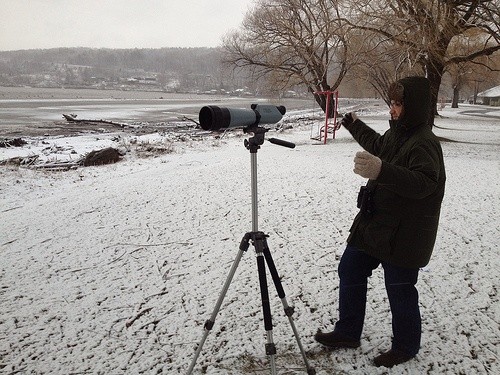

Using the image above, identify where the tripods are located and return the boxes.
[186,128,314,375]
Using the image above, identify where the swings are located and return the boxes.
[309,94,342,142]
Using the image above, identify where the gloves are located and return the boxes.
[352,150,382,180]
[340,111,359,129]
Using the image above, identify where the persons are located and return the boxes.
[313,76,447,368]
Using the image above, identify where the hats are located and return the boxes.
[387,75,439,107]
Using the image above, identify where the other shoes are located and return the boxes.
[373,349,412,367]
[314,330,361,348]
